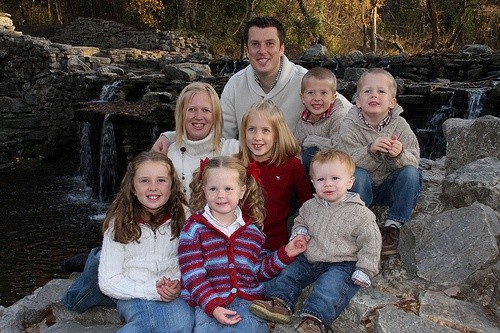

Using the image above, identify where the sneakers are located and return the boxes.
[382,224,401,255]
[249,298,290,324]
[294,315,334,333]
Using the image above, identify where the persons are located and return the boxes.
[165,83,240,203]
[151,16,355,155]
[178,156,310,333]
[339,68,420,254]
[249,149,382,333]
[98,152,194,333]
[294,66,346,172]
[232,100,312,250]
